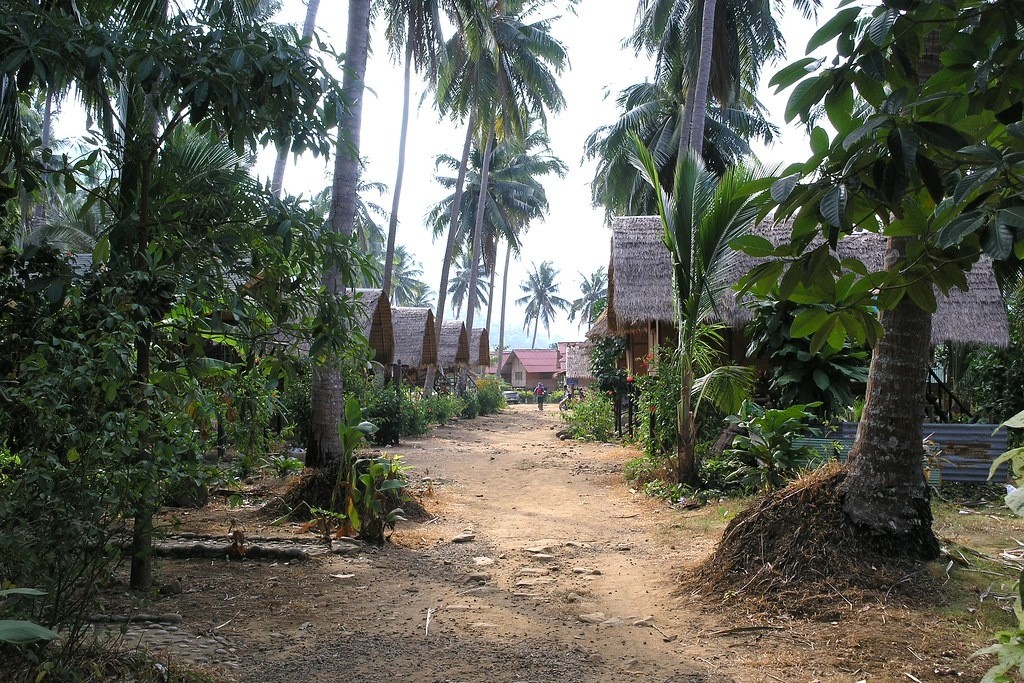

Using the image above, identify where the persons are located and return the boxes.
[563,385,577,400]
[533,382,546,411]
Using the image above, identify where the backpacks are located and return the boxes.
[537,387,543,395]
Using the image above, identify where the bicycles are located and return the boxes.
[559,385,586,412]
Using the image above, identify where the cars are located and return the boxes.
[503,391,520,404]
